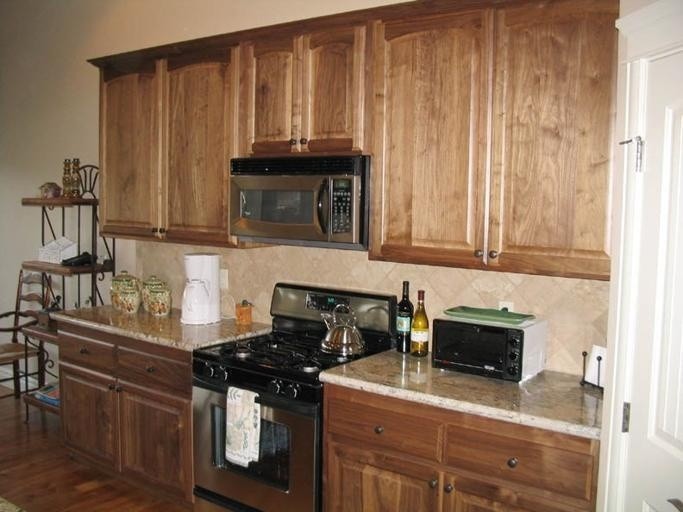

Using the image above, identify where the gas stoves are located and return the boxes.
[191,331,379,403]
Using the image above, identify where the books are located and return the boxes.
[35,380,60,407]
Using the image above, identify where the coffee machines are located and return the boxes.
[180,252,221,324]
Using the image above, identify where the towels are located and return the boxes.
[224,385,262,470]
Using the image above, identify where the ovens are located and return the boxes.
[190,373,321,512]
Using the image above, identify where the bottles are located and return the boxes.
[61,159,70,199]
[409,290,429,357]
[110,269,171,317]
[395,279,414,354]
[408,358,428,395]
[238,299,252,325]
[70,158,80,198]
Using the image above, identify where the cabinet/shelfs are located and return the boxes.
[320,381,596,512]
[85,31,281,248]
[57,318,194,503]
[366,2,608,280]
[237,8,364,159]
[20,184,117,429]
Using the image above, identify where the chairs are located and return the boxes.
[0,267,51,403]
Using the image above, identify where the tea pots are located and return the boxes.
[319,304,367,354]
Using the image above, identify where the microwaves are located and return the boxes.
[431,305,545,384]
[229,153,371,253]
